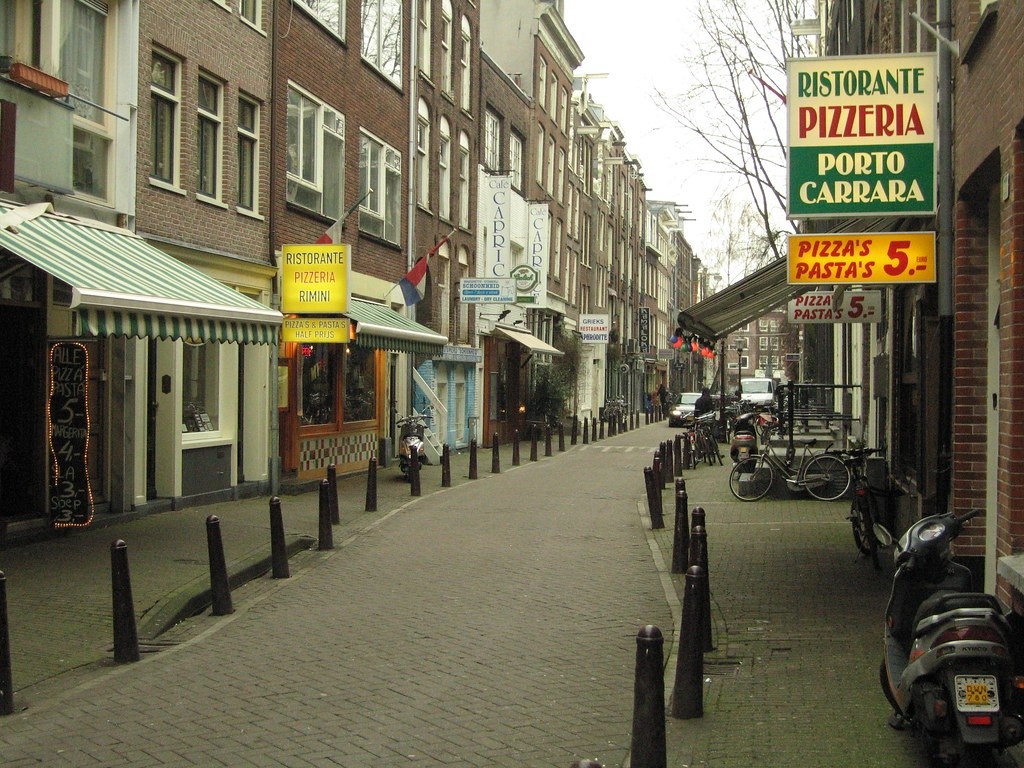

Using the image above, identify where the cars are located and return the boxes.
[667,391,702,428]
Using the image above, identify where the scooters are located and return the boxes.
[392,404,435,484]
[870,508,1024,768]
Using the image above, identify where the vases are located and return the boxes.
[0,54,70,99]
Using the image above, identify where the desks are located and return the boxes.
[770,418,840,460]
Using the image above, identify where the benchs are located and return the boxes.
[847,436,857,443]
[739,444,771,497]
[830,426,840,431]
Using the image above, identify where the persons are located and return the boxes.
[692,386,713,426]
[657,384,667,406]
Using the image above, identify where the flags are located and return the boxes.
[314,195,366,246]
[398,237,448,306]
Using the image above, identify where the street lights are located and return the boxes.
[737,348,743,401]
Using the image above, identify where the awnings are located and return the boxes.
[0,203,283,347]
[679,218,906,343]
[493,327,564,357]
[341,297,448,357]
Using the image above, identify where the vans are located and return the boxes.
[735,377,775,407]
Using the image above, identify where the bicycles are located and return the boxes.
[602,395,630,422]
[823,439,885,570]
[681,394,788,473]
[727,413,852,502]
